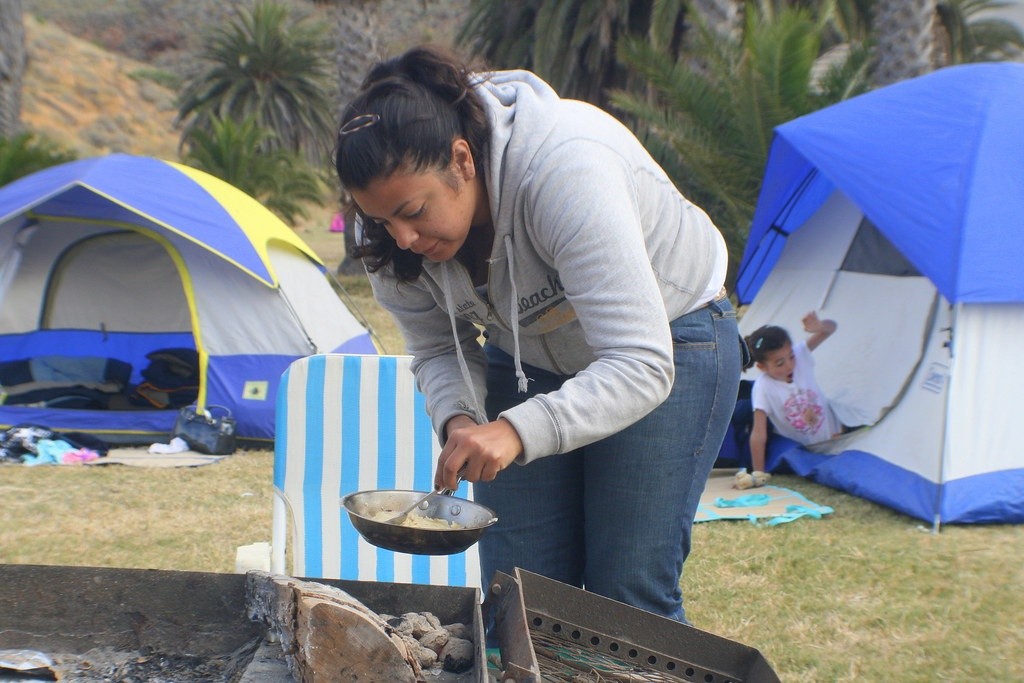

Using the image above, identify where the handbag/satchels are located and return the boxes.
[171,404,237,455]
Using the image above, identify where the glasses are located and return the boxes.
[755,324,776,361]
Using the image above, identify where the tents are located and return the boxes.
[713,58,1024,535]
[0,154,388,453]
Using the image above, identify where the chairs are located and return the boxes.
[270,353,485,602]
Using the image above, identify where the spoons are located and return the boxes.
[384,490,438,525]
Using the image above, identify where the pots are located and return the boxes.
[341,468,498,555]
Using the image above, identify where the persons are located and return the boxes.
[743,312,845,491]
[334,43,751,648]
